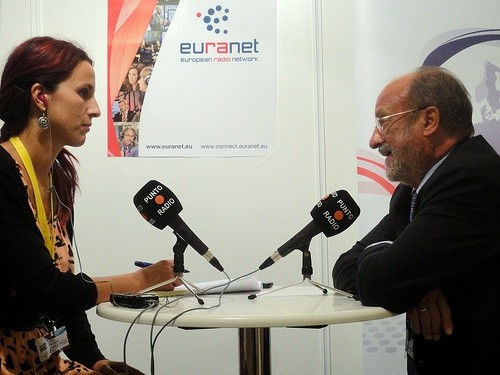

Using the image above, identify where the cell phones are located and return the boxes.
[110,292,159,307]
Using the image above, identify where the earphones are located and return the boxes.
[38,92,48,106]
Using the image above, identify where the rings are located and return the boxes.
[419,308,427,313]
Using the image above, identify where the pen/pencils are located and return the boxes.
[135,261,190,273]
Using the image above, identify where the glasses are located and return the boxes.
[375,105,426,136]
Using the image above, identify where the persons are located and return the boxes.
[0,37,184,375]
[114,40,161,156]
[331,68,500,375]
[149,6,167,30]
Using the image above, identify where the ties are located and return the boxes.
[123,113,126,122]
[125,147,130,155]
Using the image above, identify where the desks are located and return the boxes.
[97,281,401,375]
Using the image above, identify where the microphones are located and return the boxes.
[260,190,360,270]
[133,180,225,272]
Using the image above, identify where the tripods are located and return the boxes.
[248,236,360,300]
[138,230,208,305]
